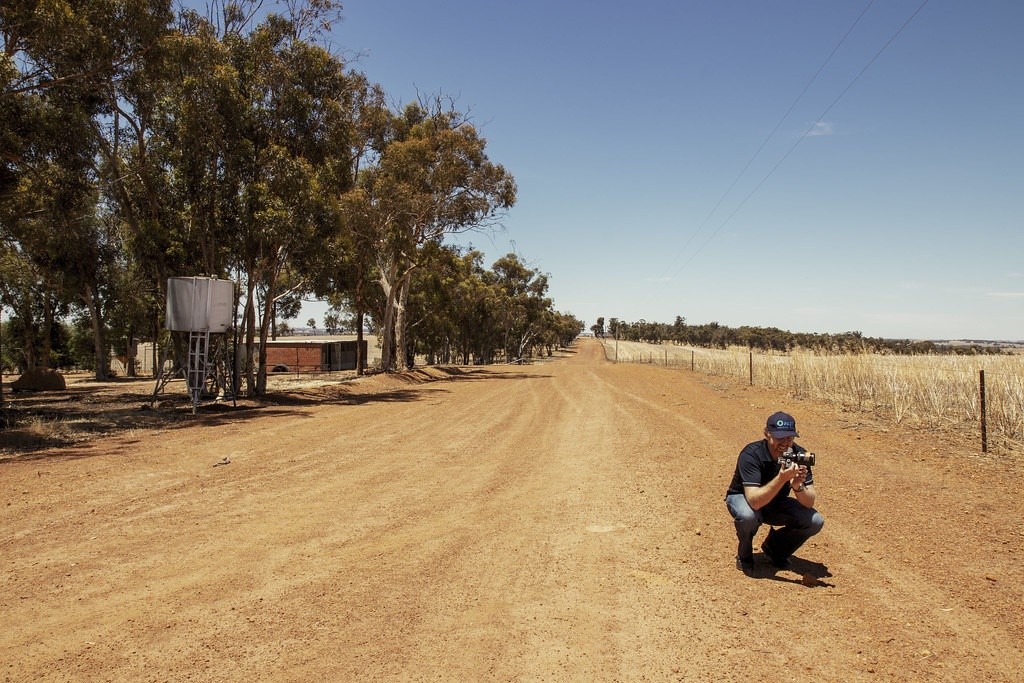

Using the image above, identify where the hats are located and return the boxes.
[767,411,799,439]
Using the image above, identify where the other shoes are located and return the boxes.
[761,543,791,568]
[735,551,753,571]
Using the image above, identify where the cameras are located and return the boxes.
[778,447,816,469]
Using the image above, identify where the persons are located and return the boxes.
[723,410,824,572]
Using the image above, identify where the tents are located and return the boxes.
[11,366,66,392]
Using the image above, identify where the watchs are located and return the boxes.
[791,482,804,492]
